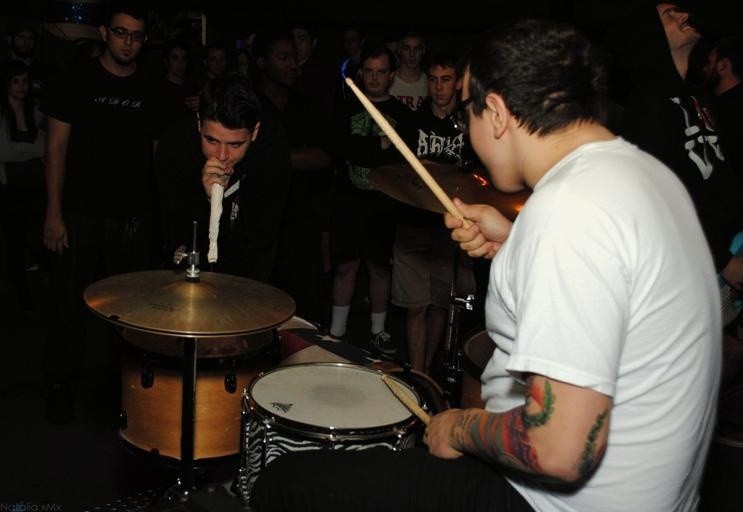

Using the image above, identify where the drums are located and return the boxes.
[459,329,497,409]
[368,362,451,414]
[238,363,421,501]
[117,328,283,462]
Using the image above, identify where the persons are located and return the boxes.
[0,1,742,377]
[246,17,727,512]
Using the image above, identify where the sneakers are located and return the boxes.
[316,328,343,343]
[370,331,397,353]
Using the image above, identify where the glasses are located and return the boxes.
[447,98,475,132]
[112,27,149,43]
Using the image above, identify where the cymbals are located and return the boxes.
[83,269,296,339]
[373,161,533,221]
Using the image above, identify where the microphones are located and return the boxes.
[207,171,228,265]
